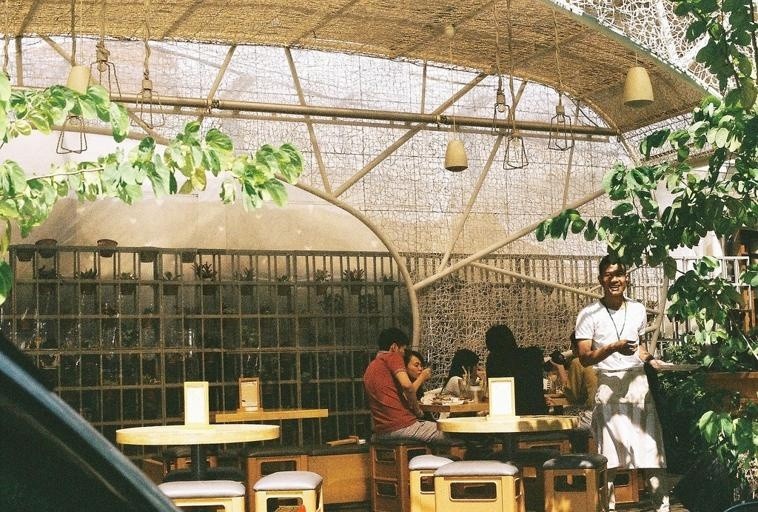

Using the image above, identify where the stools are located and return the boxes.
[407,452,453,511]
[432,459,526,511]
[368,438,432,511]
[253,469,325,511]
[588,437,641,504]
[157,479,246,512]
[541,452,608,509]
[143,437,371,503]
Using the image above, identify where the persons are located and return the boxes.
[573,253,678,511]
[363,326,470,485]
[440,325,597,413]
[401,344,432,410]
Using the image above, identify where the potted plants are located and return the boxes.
[0,239,413,470]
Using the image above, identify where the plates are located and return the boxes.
[659,364,698,371]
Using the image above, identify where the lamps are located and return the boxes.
[623,52,655,109]
[445,24,468,173]
[548,6,575,151]
[503,0,529,170]
[491,1,510,136]
[56,0,165,155]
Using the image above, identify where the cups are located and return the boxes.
[458,379,471,400]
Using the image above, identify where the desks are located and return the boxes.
[437,416,574,463]
[210,408,328,449]
[420,395,568,418]
[115,425,280,480]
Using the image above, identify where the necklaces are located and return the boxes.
[601,296,626,343]
